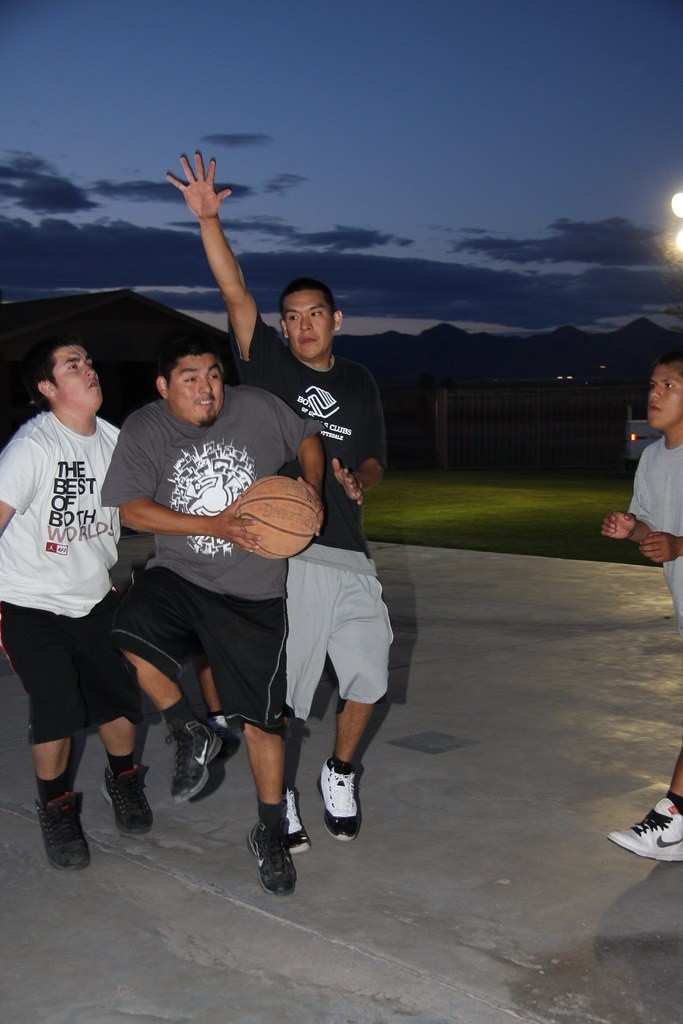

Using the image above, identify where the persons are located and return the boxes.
[601,350,683,861]
[0,336,153,871]
[101,338,325,896]
[167,151,393,855]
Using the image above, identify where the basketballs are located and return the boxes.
[235,476,318,560]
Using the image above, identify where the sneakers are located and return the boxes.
[246,821,297,897]
[317,757,361,841]
[606,798,683,861]
[100,763,154,835]
[165,717,224,802]
[282,787,311,854]
[204,711,232,749]
[35,791,90,870]
